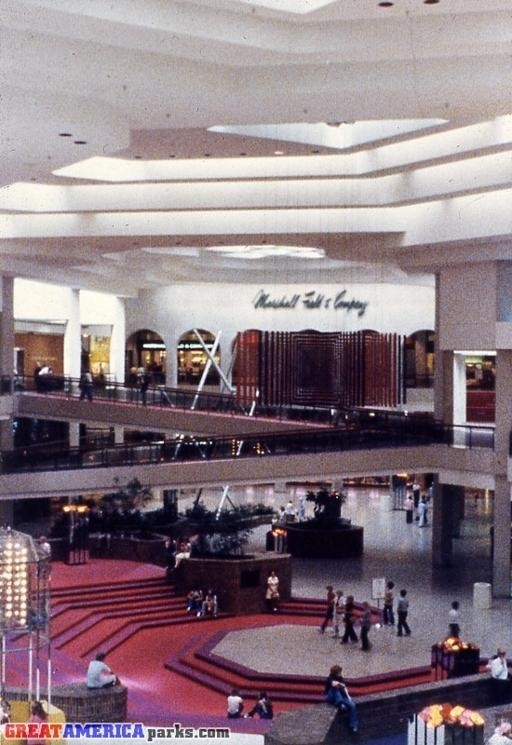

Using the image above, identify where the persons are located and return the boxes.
[243,690,274,720]
[490,647,508,706]
[265,570,280,612]
[319,578,412,651]
[26,700,49,745]
[166,537,177,569]
[200,588,218,617]
[38,535,51,555]
[324,664,359,736]
[227,689,244,719]
[174,537,192,568]
[271,497,305,525]
[86,652,116,690]
[448,601,461,639]
[186,589,203,617]
[488,722,512,745]
[34,361,149,408]
[404,479,429,528]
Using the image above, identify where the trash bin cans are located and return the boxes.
[472,582,492,609]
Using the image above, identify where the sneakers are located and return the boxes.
[340,703,358,734]
[187,606,218,618]
[243,712,253,720]
[316,627,374,651]
